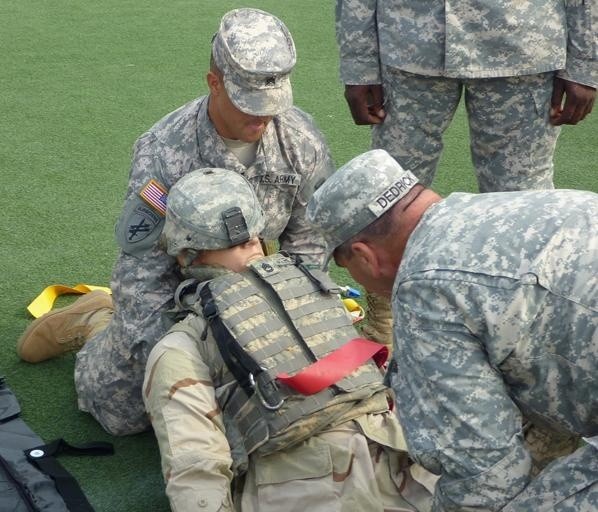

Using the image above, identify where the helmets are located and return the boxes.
[162,168,265,257]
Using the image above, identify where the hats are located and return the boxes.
[211,6,297,117]
[304,149,421,273]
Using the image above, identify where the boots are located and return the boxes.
[15,288,120,364]
[360,291,392,360]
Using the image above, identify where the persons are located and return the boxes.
[139,166,441,512]
[333,0,597,369]
[304,148,597,512]
[15,6,335,438]
